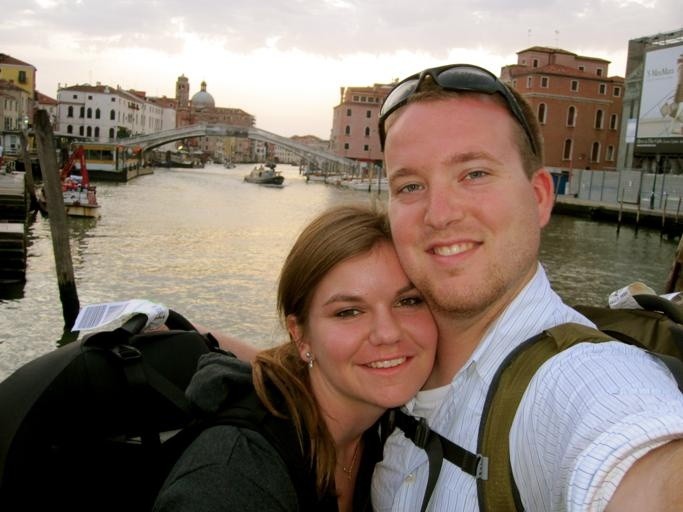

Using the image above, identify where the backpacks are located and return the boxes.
[1,309,397,512]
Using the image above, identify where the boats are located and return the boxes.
[241,165,285,185]
[29,142,102,219]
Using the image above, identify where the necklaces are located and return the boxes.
[337,443,360,481]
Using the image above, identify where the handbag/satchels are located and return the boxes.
[466,294,680,511]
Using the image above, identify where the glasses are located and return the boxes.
[378,64,536,157]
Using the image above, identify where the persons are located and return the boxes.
[152,202,438,512]
[142,65,683,512]
